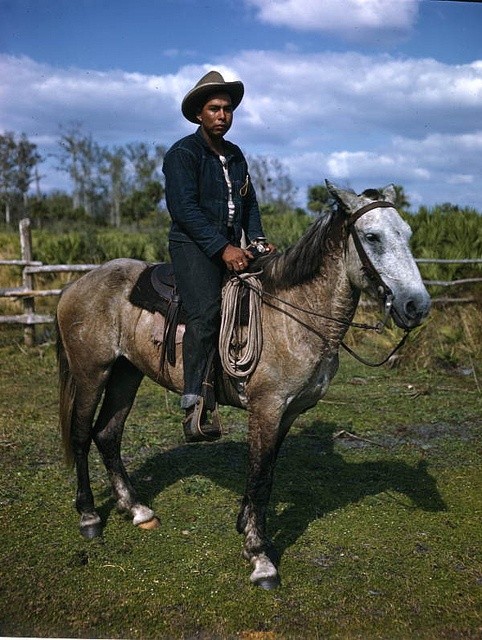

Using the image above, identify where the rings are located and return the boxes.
[237,261,243,265]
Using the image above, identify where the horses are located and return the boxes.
[54,179,432,592]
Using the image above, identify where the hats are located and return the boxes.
[182,71,244,124]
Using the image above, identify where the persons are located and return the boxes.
[160,69,277,445]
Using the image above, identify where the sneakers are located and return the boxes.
[182,417,221,443]
